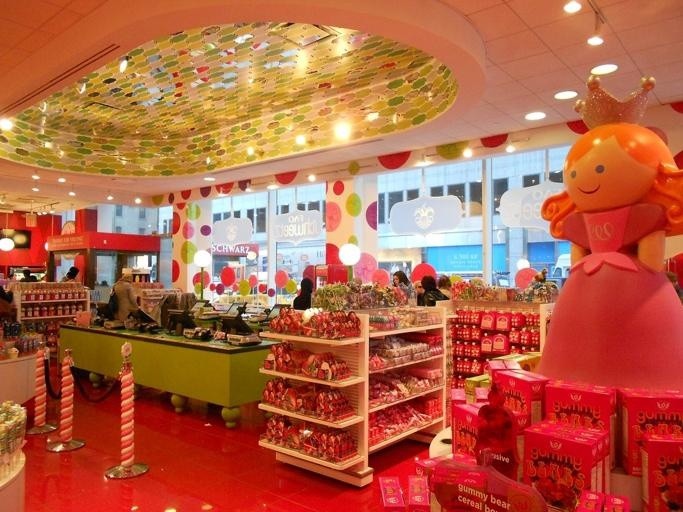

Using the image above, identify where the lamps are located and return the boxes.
[562,1,582,14]
[524,111,547,121]
[589,63,618,76]
[553,89,578,100]
[586,35,604,47]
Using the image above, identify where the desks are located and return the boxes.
[58,322,281,428]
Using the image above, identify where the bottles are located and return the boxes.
[21,289,26,301]
[38,320,46,331]
[57,304,62,315]
[68,290,73,299]
[64,304,69,315]
[49,304,54,315]
[46,320,55,334]
[78,303,82,311]
[71,304,76,314]
[34,305,40,316]
[61,289,65,299]
[48,332,56,347]
[39,289,43,301]
[41,305,47,315]
[27,306,32,317]
[80,288,85,298]
[21,305,25,317]
[29,289,35,300]
[54,290,59,300]
[74,289,79,298]
[45,290,50,299]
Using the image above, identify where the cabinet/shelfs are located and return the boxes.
[13,288,90,351]
[257,305,447,488]
[437,299,555,395]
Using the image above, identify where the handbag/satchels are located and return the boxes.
[109,287,117,312]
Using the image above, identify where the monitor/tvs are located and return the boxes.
[226,302,247,316]
[133,308,155,323]
[189,299,209,313]
[266,303,291,320]
[222,315,254,334]
[167,309,197,328]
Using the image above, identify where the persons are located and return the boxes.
[60,267,79,282]
[38,273,48,282]
[413,280,425,306]
[666,271,682,298]
[21,269,37,283]
[438,275,453,299]
[112,273,138,320]
[0,285,14,322]
[421,277,449,306]
[294,278,313,310]
[391,271,409,287]
